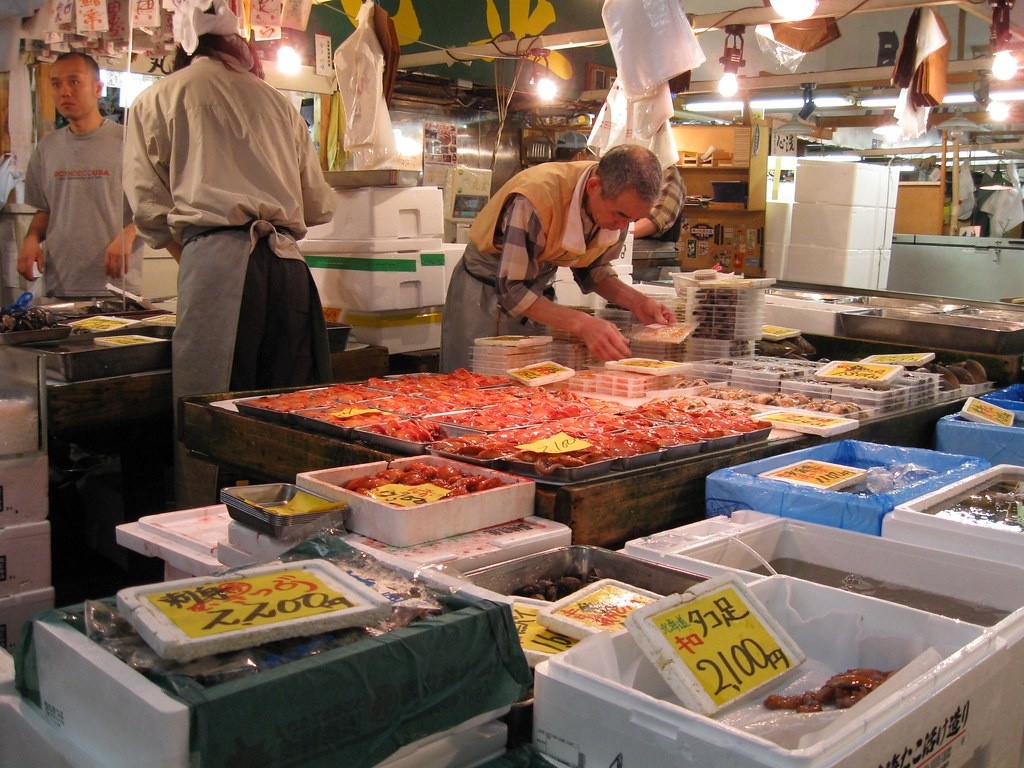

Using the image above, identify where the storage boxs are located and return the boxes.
[0,384,1023,767]
[296,185,468,353]
[553,222,635,309]
[764,158,899,289]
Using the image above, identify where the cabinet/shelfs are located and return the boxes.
[671,122,770,277]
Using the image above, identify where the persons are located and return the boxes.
[122,0,338,511]
[17,51,142,302]
[551,131,686,252]
[440,143,678,375]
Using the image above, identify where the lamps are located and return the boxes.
[774,112,815,136]
[798,83,817,120]
[528,47,558,100]
[973,68,1009,121]
[718,24,745,96]
[935,106,979,137]
[987,1,1017,81]
[979,163,1013,191]
